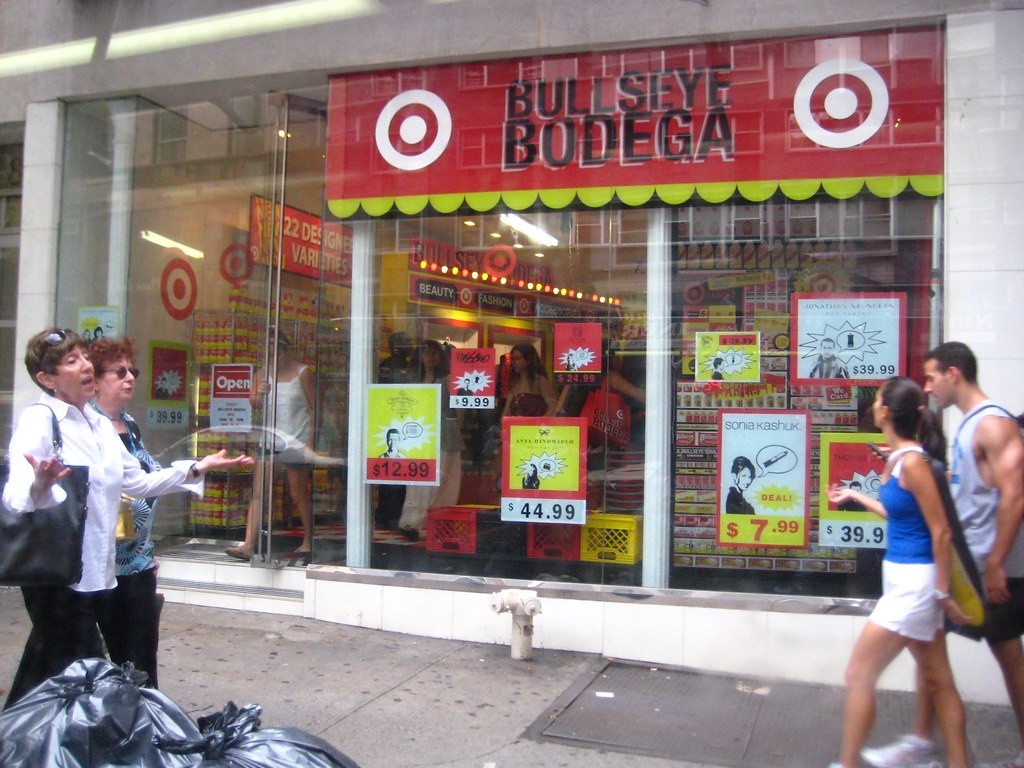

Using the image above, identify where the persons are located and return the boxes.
[499,345,559,416]
[398,341,465,537]
[1,328,256,715]
[380,332,414,532]
[82,336,163,694]
[225,325,324,561]
[825,377,975,768]
[553,357,646,510]
[861,341,1024,768]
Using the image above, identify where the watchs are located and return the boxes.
[934,588,952,601]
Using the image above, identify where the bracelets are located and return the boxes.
[191,463,200,479]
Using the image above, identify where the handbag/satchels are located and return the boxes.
[580,372,632,448]
[929,459,988,627]
[0,403,90,586]
[116,493,135,542]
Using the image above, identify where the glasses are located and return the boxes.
[37,329,76,368]
[104,366,139,379]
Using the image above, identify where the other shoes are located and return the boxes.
[396,526,419,543]
[976,748,1024,768]
[861,733,940,768]
[225,546,250,560]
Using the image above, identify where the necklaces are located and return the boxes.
[89,398,125,422]
